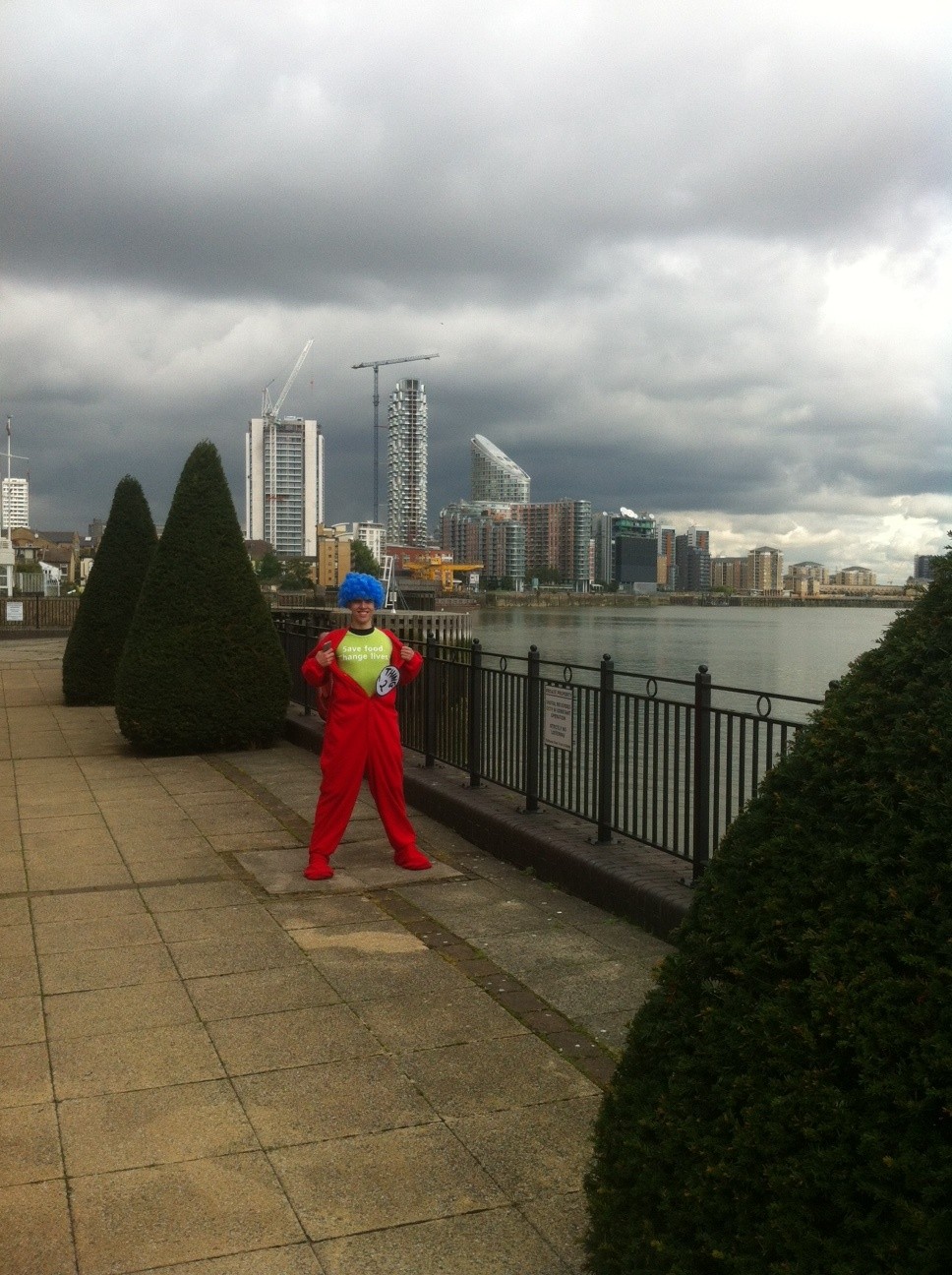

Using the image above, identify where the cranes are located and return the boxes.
[349,354,440,524]
[259,336,317,421]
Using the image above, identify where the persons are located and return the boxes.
[302,573,432,879]
